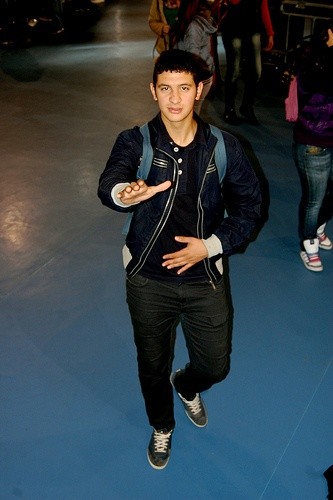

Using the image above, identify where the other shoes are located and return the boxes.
[224,108,235,121]
[240,108,258,123]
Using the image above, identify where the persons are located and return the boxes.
[147,0,213,117]
[219,0,274,126]
[293,18,333,273]
[97,49,263,471]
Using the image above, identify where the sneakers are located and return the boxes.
[314,222,332,250]
[171,369,208,428]
[300,239,323,271]
[147,419,175,469]
